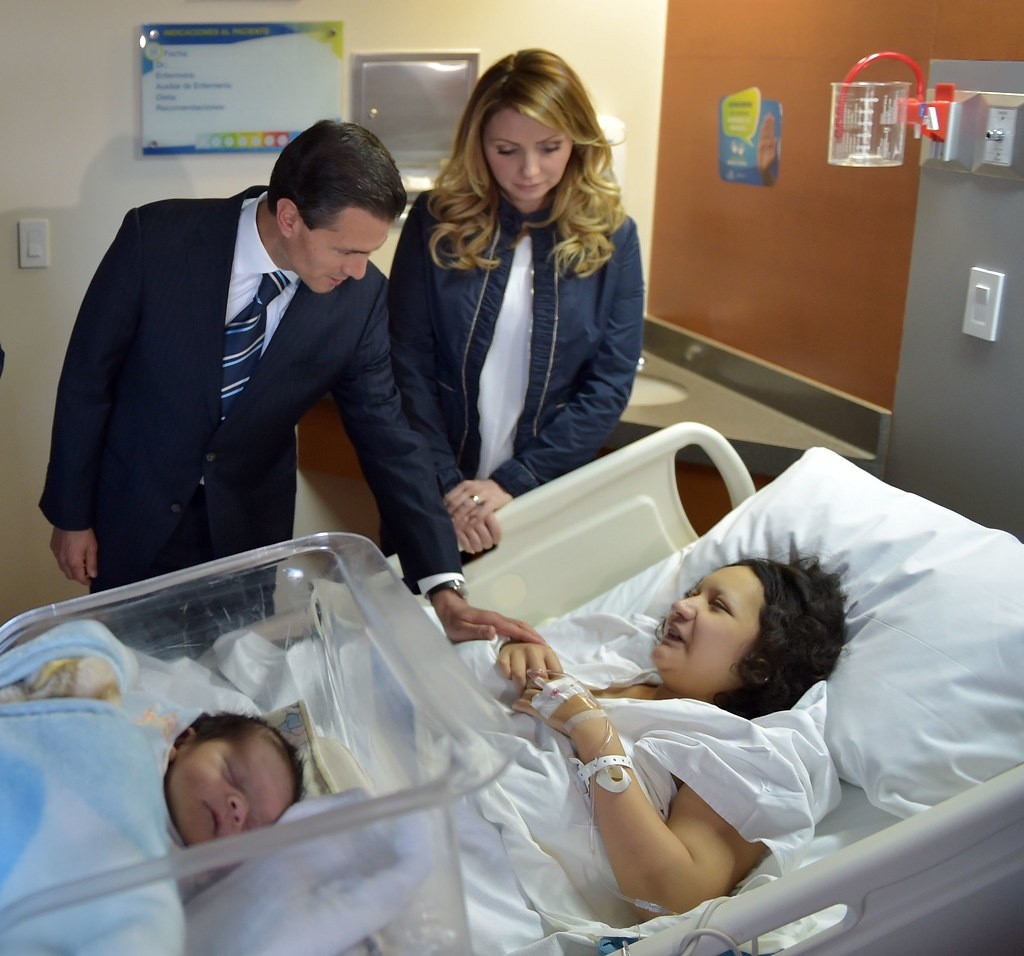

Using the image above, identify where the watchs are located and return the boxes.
[429,577,469,604]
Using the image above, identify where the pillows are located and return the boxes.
[651,446,1024,818]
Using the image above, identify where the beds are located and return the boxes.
[190,417,1024,954]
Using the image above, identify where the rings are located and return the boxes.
[468,493,479,505]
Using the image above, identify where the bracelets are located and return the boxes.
[570,753,635,793]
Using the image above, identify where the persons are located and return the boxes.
[39,120,546,665]
[1,692,304,956]
[339,558,847,956]
[387,49,645,554]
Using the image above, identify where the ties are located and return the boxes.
[221,271,291,423]
